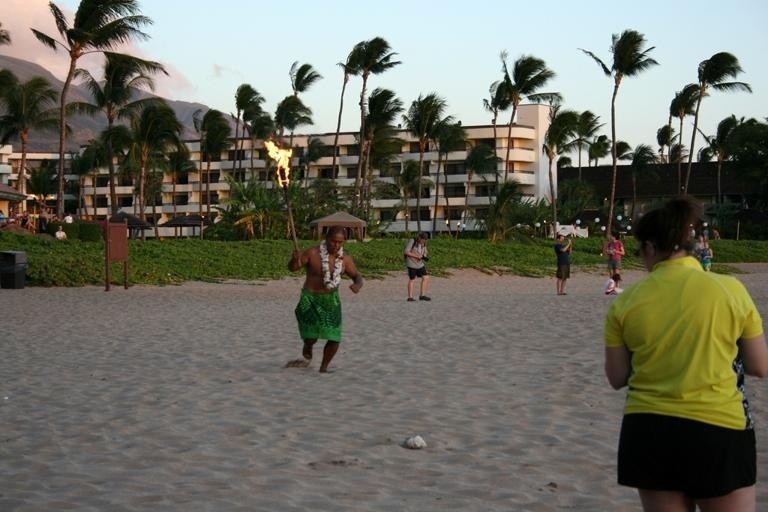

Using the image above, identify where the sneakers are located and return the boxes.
[408,298,415,301]
[419,296,430,301]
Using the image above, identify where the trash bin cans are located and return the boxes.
[0,250,28,289]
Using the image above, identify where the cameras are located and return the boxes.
[421,254,429,262]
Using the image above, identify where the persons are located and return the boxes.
[0,204,74,242]
[403,232,433,301]
[712,229,721,240]
[287,228,363,373]
[604,197,767,512]
[688,224,695,239]
[692,234,705,262]
[603,232,625,285]
[605,274,625,295]
[554,235,572,296]
[700,242,714,272]
